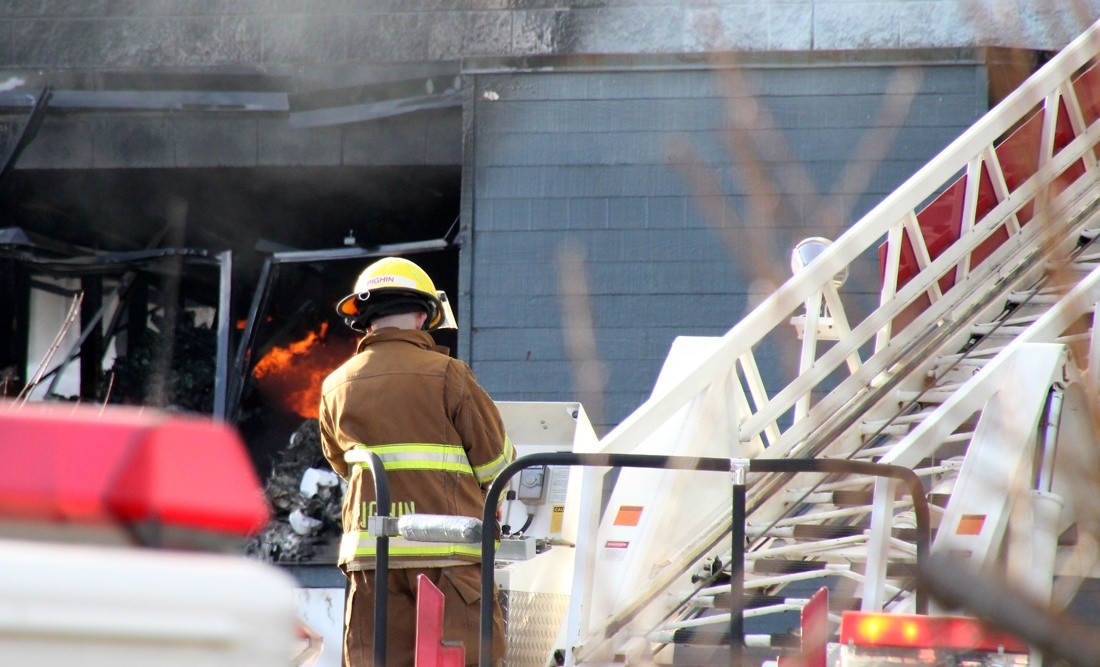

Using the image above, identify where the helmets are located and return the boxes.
[336,257,446,332]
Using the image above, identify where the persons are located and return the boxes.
[318,257,516,667]
